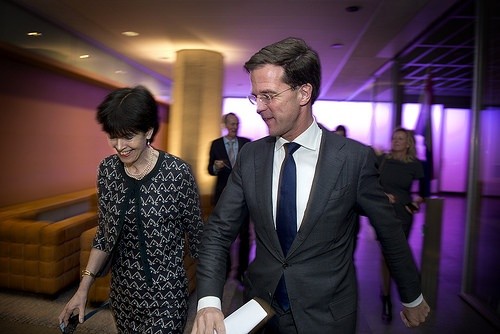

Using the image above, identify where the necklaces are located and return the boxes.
[124,145,154,180]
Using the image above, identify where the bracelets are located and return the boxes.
[81,269,96,280]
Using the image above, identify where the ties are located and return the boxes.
[228,142,236,168]
[272,143,302,312]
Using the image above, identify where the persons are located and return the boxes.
[377,128,428,324]
[189,37,431,334]
[332,124,348,137]
[206,112,252,282]
[59,85,205,334]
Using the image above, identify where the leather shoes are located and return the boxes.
[380,292,392,322]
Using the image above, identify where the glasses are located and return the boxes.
[248,82,307,106]
